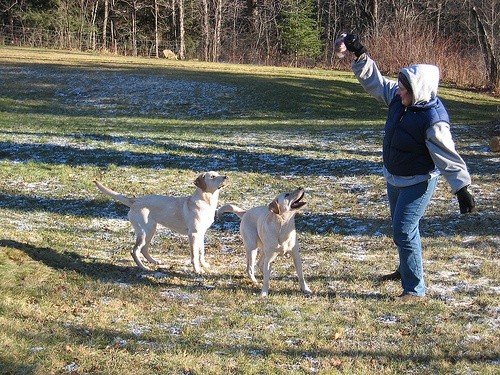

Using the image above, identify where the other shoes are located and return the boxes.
[399,293,420,301]
[384,271,401,280]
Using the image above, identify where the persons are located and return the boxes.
[337,32,475,299]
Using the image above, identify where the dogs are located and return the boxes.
[217,185,314,298]
[93,170,230,275]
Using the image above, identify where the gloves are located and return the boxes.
[458,188,475,214]
[344,34,366,56]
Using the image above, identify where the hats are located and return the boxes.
[399,73,411,92]
